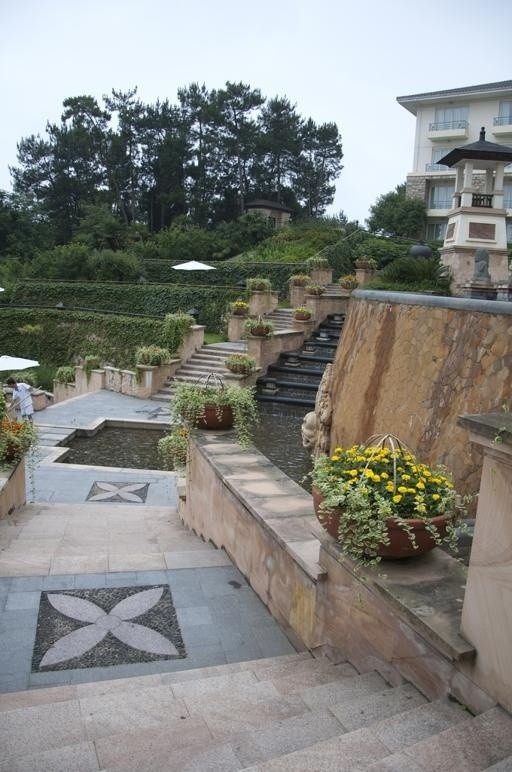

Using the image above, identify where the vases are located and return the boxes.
[0,440,31,464]
[293,311,311,319]
[231,307,249,316]
[341,282,358,290]
[310,484,453,562]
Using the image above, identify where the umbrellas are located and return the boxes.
[0,355,41,372]
[170,259,216,285]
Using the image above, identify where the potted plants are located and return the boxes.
[244,314,273,336]
[135,344,170,383]
[288,255,330,295]
[355,255,377,270]
[157,372,261,478]
[247,278,271,291]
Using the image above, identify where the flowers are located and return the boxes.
[339,274,358,291]
[299,441,480,606]
[229,301,252,315]
[295,306,313,317]
[0,414,44,506]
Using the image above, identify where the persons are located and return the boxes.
[6,377,36,430]
